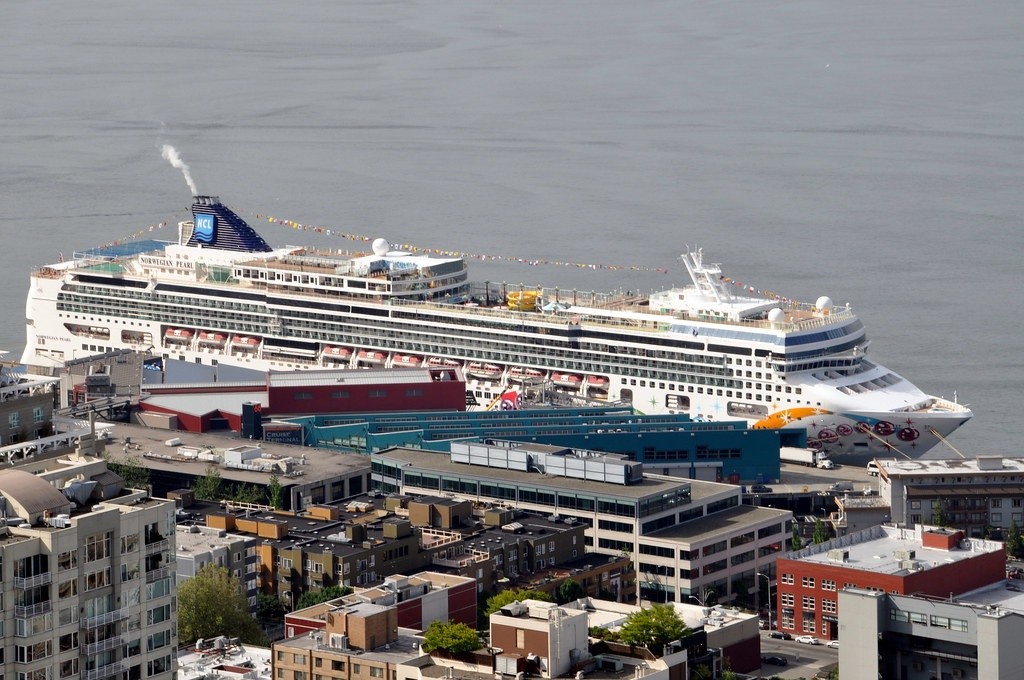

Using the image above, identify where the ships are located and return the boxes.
[20,195,973,467]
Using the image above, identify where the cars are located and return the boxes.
[794,635,820,645]
[768,631,792,640]
[825,640,839,649]
[761,655,788,667]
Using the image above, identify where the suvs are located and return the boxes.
[759,619,776,630]
[751,483,773,494]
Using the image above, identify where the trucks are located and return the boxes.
[829,481,855,492]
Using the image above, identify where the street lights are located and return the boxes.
[757,572,771,630]
